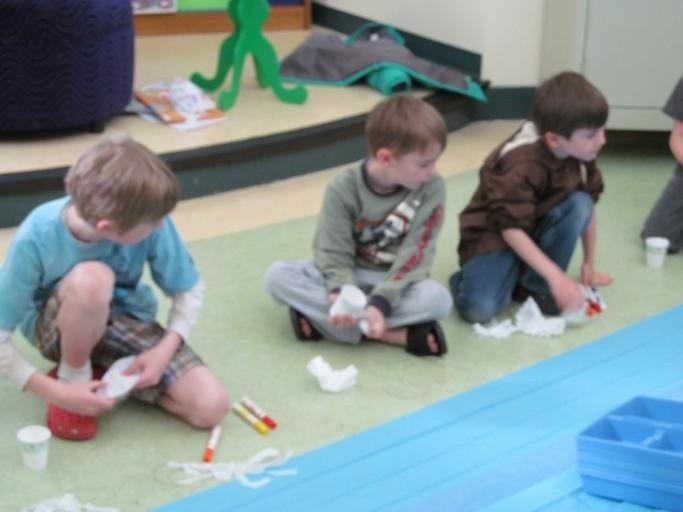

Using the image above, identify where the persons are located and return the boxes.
[267,94,453,357]
[451,69,618,324]
[0,138,229,440]
[641,77,683,253]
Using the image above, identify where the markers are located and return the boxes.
[232,403,268,433]
[583,286,604,315]
[240,396,277,429]
[203,425,221,462]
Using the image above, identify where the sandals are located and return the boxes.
[405,320,447,357]
[288,306,325,342]
[46,366,107,381]
[46,403,98,441]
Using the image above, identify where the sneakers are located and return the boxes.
[511,280,559,316]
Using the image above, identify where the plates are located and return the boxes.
[94,355,140,401]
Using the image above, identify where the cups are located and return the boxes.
[643,236,669,268]
[16,424,52,471]
[327,283,367,323]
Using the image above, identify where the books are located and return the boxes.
[133,79,229,126]
